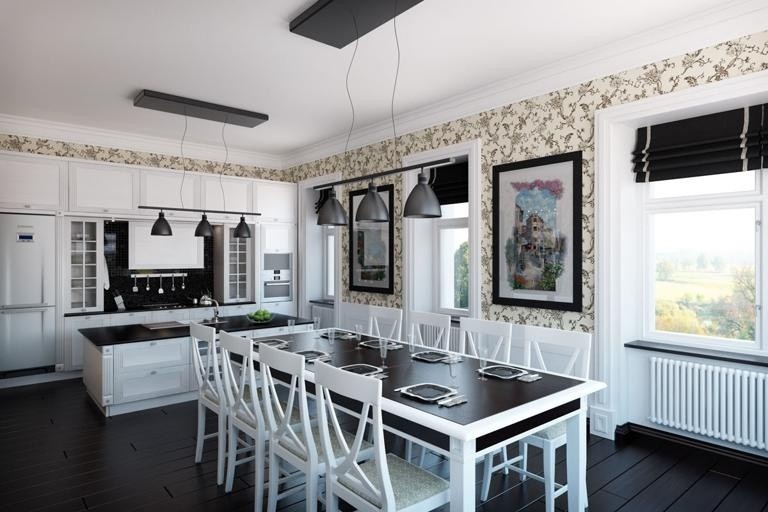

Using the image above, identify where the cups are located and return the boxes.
[287,314,490,383]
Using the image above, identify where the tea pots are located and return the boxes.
[200,288,213,306]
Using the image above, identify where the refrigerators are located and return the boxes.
[0,212,56,369]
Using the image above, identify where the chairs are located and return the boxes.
[369,306,402,342]
[480,324,592,512]
[313,360,451,510]
[418,317,512,476]
[405,310,451,349]
[258,343,375,510]
[190,320,318,512]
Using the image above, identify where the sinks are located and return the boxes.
[179,319,230,327]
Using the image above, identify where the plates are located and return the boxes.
[475,364,529,379]
[400,377,455,402]
[253,338,287,350]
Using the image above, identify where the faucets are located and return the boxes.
[202,298,221,319]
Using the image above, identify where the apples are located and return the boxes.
[249,309,270,320]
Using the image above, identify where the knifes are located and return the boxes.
[112,288,120,297]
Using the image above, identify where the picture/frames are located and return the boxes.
[348,183,394,296]
[491,151,582,312]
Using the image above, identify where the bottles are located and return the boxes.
[193,298,197,305]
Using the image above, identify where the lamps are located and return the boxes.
[289,0,442,228]
[131,88,268,239]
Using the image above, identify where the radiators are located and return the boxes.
[646,356,766,452]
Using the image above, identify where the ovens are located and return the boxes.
[259,252,293,303]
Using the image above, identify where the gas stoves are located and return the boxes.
[140,300,188,310]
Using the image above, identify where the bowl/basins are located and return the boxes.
[246,313,275,324]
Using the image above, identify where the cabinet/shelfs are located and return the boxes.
[259,223,299,300]
[116,338,191,416]
[64,215,105,315]
[214,226,251,303]
